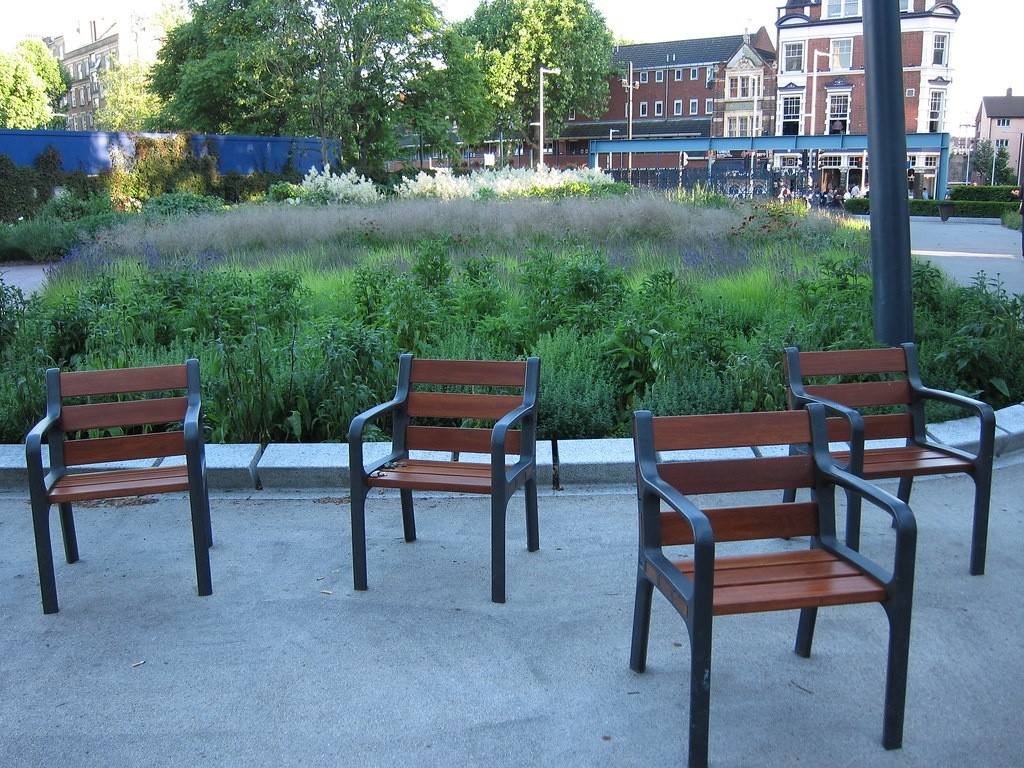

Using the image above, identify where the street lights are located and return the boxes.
[1001,130,1023,186]
[540,67,561,169]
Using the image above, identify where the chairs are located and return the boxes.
[24,358,213,615]
[629,402,917,768]
[348,353,541,603]
[782,343,996,576]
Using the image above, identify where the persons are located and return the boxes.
[921,188,928,199]
[822,187,850,209]
[784,188,802,201]
[971,180,977,186]
[851,183,869,198]
[945,187,951,199]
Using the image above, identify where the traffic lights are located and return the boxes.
[857,157,862,167]
